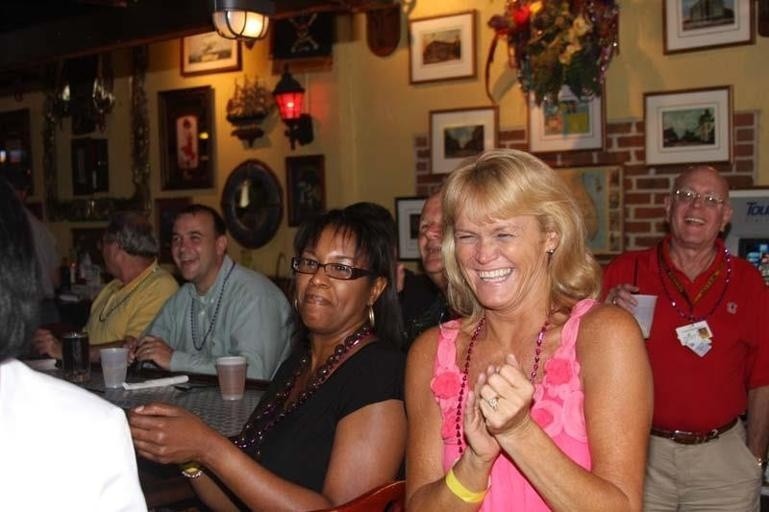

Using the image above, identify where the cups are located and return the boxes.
[215,355,248,401]
[630,295,658,340]
[100,348,129,388]
[62,332,91,382]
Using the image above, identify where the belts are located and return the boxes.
[650,417,737,446]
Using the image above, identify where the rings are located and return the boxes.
[489,398,503,410]
[146,348,150,352]
[609,297,617,309]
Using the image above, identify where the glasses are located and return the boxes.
[291,256,371,281]
[676,185,725,208]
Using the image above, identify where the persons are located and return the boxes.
[130,204,404,512]
[404,148,652,512]
[596,162,769,510]
[403,187,467,335]
[1,181,148,512]
[0,162,63,301]
[127,203,298,381]
[31,210,180,367]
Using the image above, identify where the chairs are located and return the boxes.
[314,481,406,512]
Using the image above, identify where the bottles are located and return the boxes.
[59,250,91,290]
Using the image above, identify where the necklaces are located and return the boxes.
[99,269,154,321]
[646,243,735,321]
[218,323,391,451]
[454,306,558,476]
[190,260,235,351]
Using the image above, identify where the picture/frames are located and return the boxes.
[158,85,215,191]
[428,104,500,177]
[721,188,769,285]
[527,79,606,155]
[180,32,242,76]
[396,195,430,262]
[286,154,326,229]
[661,0,759,55]
[407,8,478,85]
[643,84,735,169]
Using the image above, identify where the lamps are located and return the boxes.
[272,62,314,151]
[212,0,274,45]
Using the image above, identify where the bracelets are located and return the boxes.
[177,458,202,481]
[752,454,768,470]
[444,458,494,505]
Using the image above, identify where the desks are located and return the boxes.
[25,358,268,508]
